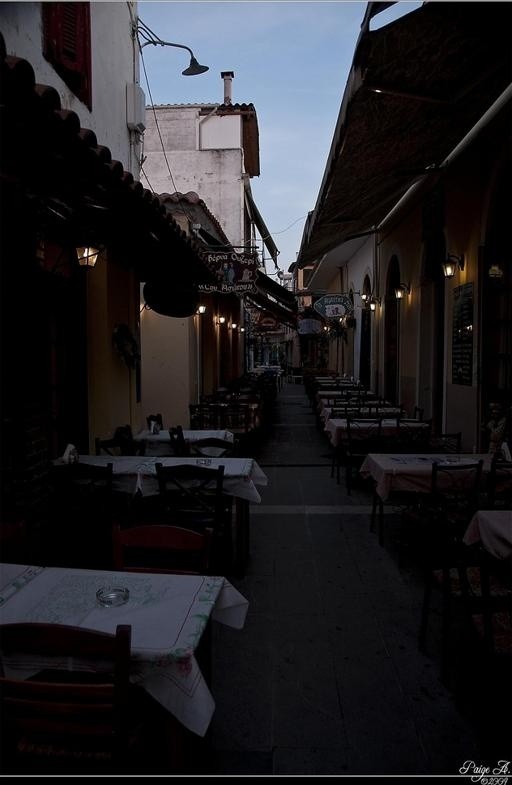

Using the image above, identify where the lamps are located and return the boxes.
[139,40,209,75]
[71,239,101,268]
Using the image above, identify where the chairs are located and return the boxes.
[303,370,511,742]
[1,362,286,775]
